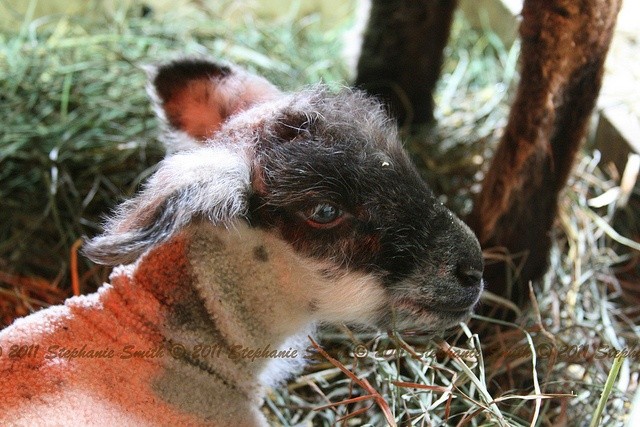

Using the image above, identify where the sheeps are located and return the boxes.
[2,56,484,427]
